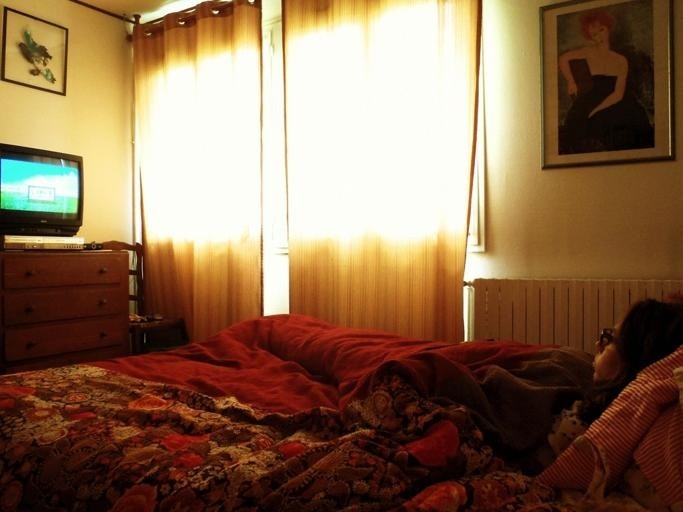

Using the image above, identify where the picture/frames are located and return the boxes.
[538,0,674,170]
[0,5,68,96]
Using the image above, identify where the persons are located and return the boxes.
[575,297,682,429]
[556,7,655,152]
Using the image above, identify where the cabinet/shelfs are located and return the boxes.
[0,250,129,374]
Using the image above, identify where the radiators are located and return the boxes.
[474,279,683,356]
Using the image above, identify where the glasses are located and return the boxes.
[600,328,624,351]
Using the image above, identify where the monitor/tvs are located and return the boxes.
[0,143,84,236]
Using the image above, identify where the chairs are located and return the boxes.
[101,240,185,357]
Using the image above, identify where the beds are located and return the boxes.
[0,315,683,511]
